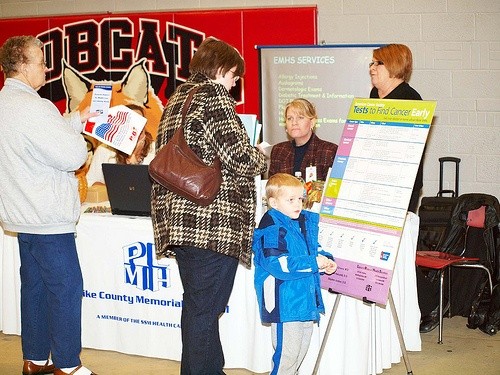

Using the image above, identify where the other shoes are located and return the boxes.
[23,359,55,375]
[54,367,97,375]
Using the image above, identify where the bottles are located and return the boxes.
[295,172,307,198]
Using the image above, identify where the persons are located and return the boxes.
[85,103,157,203]
[249,173,338,374]
[150,38,271,375]
[368,43,424,213]
[0,35,101,374]
[270,97,337,181]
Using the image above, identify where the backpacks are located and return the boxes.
[467,269,500,336]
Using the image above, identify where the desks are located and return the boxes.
[0,200,422,375]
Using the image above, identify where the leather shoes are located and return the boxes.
[419,295,451,334]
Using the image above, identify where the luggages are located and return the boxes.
[417,156,461,318]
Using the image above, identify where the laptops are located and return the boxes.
[101,163,153,217]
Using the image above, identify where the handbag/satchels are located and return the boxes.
[148,85,223,206]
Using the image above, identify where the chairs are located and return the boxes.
[416,193,500,345]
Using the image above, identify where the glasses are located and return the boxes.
[369,60,385,68]
[29,61,47,66]
[229,68,239,81]
